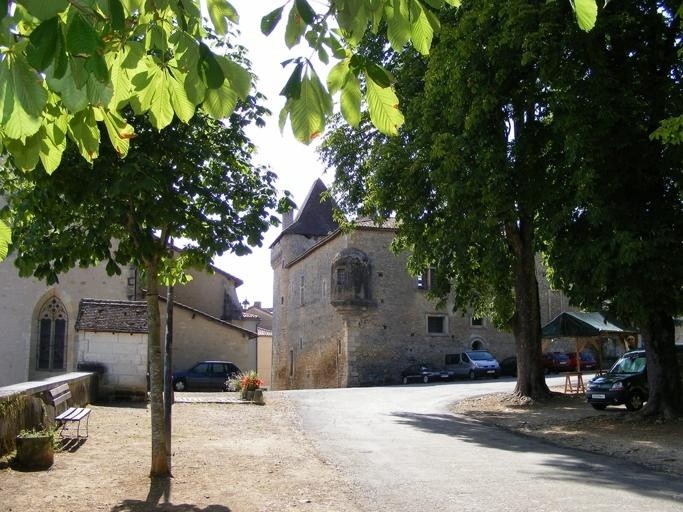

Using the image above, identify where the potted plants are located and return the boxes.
[16,427,55,469]
[224,369,264,402]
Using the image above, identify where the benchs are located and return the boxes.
[49,383,91,441]
[564,370,609,397]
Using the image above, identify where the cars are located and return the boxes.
[500,357,517,376]
[587,343,683,410]
[400,363,455,384]
[171,360,244,392]
[543,351,597,374]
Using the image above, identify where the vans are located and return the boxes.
[445,349,501,380]
